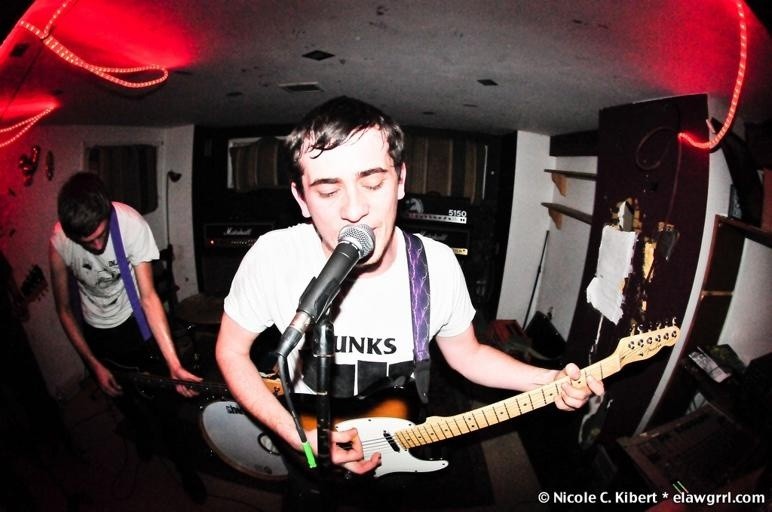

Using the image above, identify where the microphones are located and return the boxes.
[277,221,374,360]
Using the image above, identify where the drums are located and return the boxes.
[197,369,290,482]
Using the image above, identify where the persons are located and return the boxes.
[214,95,605,511]
[47,170,216,505]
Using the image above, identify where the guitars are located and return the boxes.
[86,358,234,402]
[274,317,680,501]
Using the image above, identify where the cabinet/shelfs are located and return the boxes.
[566,94,710,436]
[644,217,768,453]
[540,169,595,229]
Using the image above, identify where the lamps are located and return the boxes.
[166,169,182,246]
